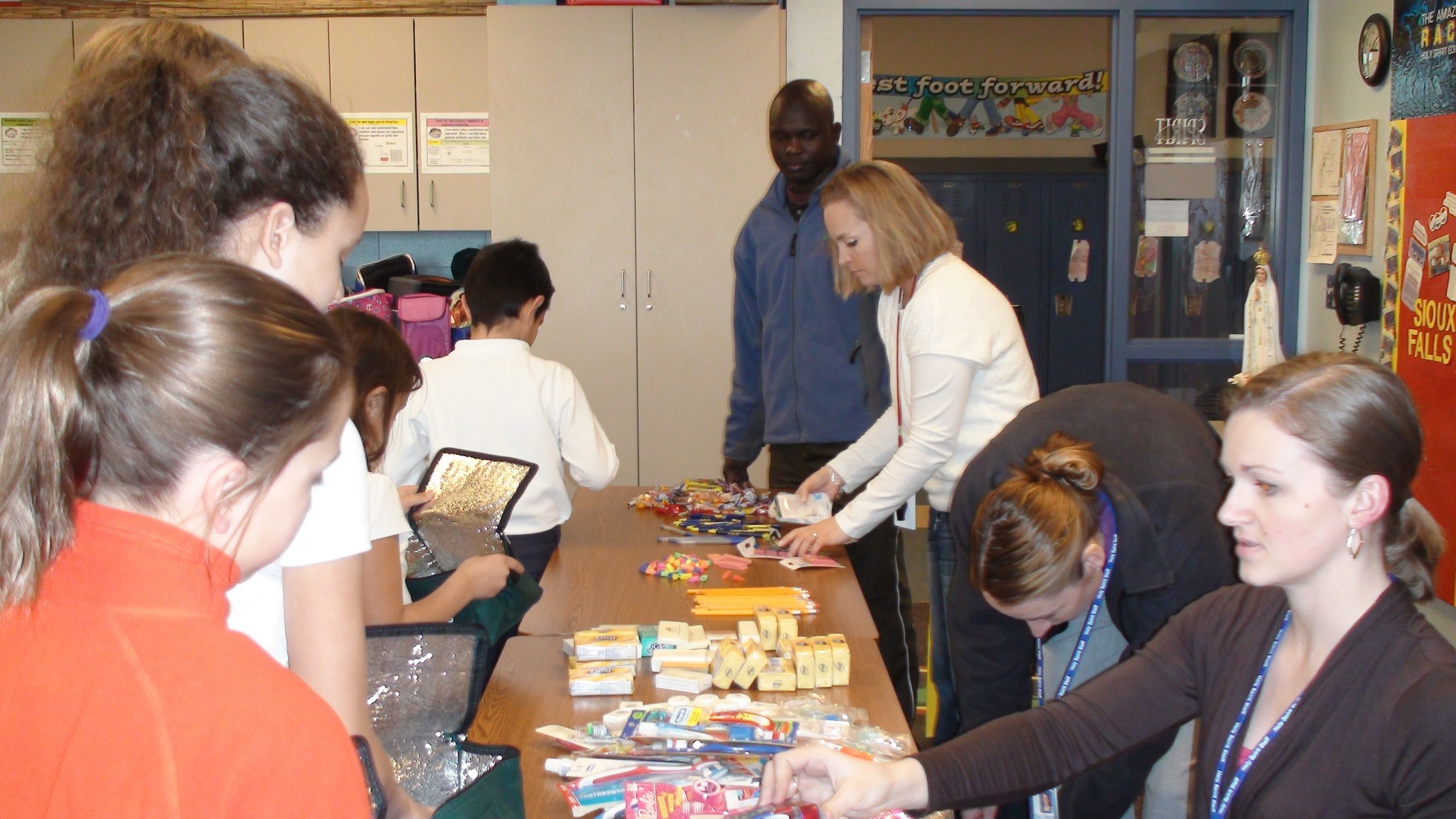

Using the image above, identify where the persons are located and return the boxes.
[720,80,918,732]
[1041,794,1053,813]
[332,308,523,624]
[0,252,374,819]
[75,17,250,84]
[379,239,621,583]
[777,160,1042,778]
[42,64,438,819]
[945,384,1240,819]
[754,355,1456,819]
[1227,264,1286,386]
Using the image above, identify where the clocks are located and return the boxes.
[1359,12,1391,86]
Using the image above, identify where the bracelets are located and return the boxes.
[826,465,843,499]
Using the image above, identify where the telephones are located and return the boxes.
[1333,262,1382,326]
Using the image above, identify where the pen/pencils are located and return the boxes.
[687,585,821,616]
[658,513,782,548]
[628,500,636,506]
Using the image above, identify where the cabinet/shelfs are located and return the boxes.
[0,19,106,231]
[328,15,490,230]
[915,171,1107,398]
[486,5,787,485]
[184,19,330,103]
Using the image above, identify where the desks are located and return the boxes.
[466,487,922,640]
[464,635,919,819]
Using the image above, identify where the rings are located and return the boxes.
[792,778,798,792]
[811,532,817,539]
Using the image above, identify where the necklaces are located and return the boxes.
[897,276,916,521]
